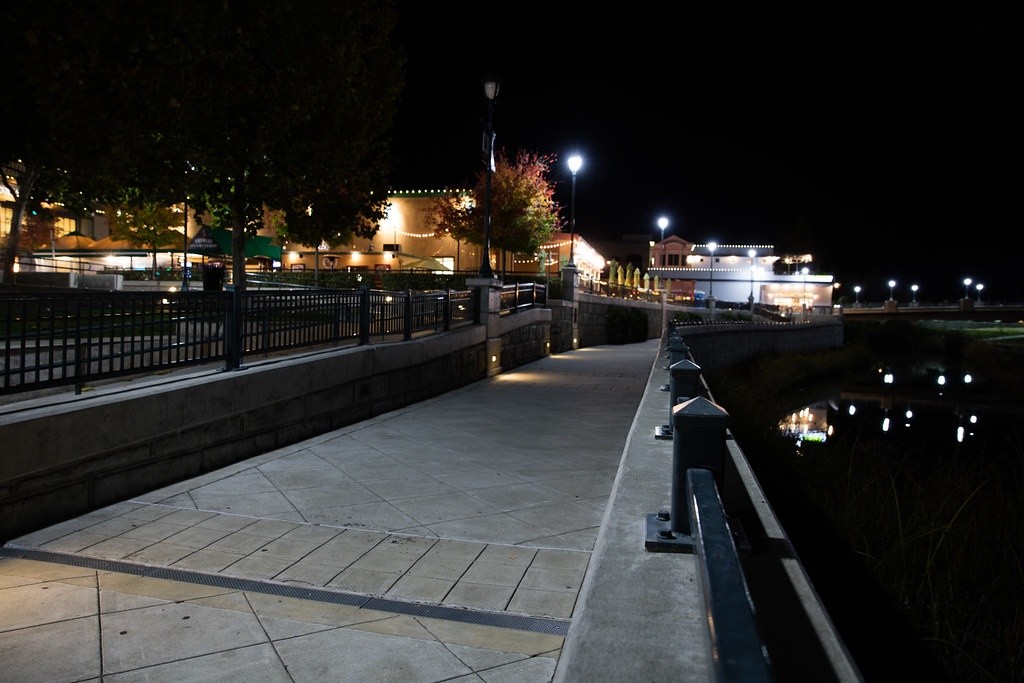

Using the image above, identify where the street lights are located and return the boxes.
[657,217,669,290]
[748,249,756,298]
[977,284,983,301]
[964,278,971,299]
[834,283,840,307]
[889,280,895,301]
[911,285,918,303]
[567,152,583,265]
[324,255,341,271]
[855,287,861,304]
[252,255,272,280]
[802,267,809,304]
[708,242,717,300]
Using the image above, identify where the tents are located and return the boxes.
[187,226,282,274]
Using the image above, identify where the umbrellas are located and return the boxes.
[643,274,651,302]
[625,261,633,299]
[631,267,640,299]
[37,230,97,274]
[151,230,193,269]
[616,265,625,299]
[608,258,616,296]
[89,228,155,271]
[654,276,661,303]
